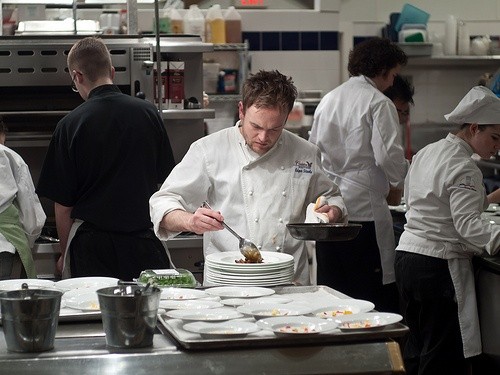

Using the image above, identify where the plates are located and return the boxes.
[157,298,224,312]
[182,321,264,338]
[256,316,336,338]
[165,305,244,321]
[221,297,294,308]
[206,285,275,300]
[236,301,313,319]
[0,278,55,291]
[204,251,295,288]
[307,297,374,319]
[56,276,122,291]
[330,312,404,332]
[61,288,101,312]
[156,286,206,300]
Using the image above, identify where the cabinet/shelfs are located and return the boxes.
[201,40,249,102]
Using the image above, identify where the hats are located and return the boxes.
[445,84,500,125]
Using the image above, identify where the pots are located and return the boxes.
[286,222,365,243]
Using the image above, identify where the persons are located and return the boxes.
[146,67,352,293]
[377,72,414,127]
[392,84,500,374]
[305,35,410,319]
[0,117,47,279]
[36,35,177,279]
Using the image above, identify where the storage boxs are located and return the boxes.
[133,268,201,288]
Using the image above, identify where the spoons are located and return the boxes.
[204,202,264,263]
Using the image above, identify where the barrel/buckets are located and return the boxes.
[0,283,70,354]
[95,285,162,349]
[162,4,244,44]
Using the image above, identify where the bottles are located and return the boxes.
[119,8,128,35]
[99,10,120,36]
[456,18,472,56]
[444,15,458,56]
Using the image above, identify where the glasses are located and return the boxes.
[69,71,82,92]
